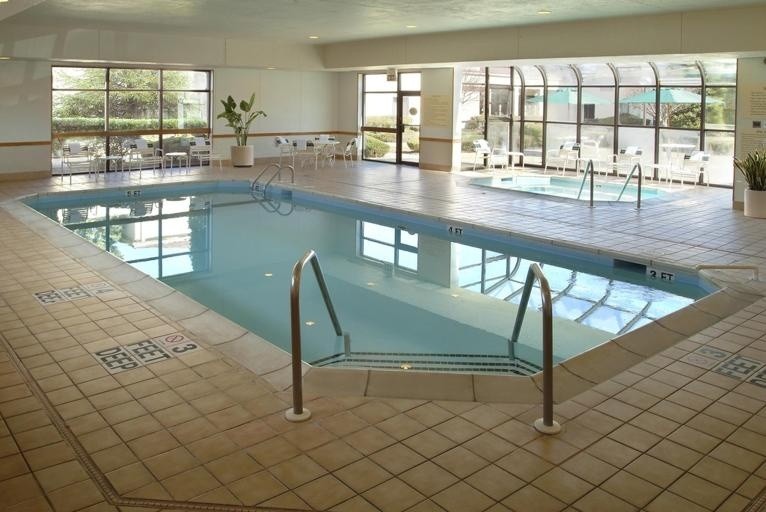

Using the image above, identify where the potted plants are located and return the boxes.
[216,93,279,167]
[734,149,766,219]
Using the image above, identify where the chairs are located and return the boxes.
[62,137,223,185]
[472,130,705,187]
[274,133,357,169]
[63,194,210,223]
